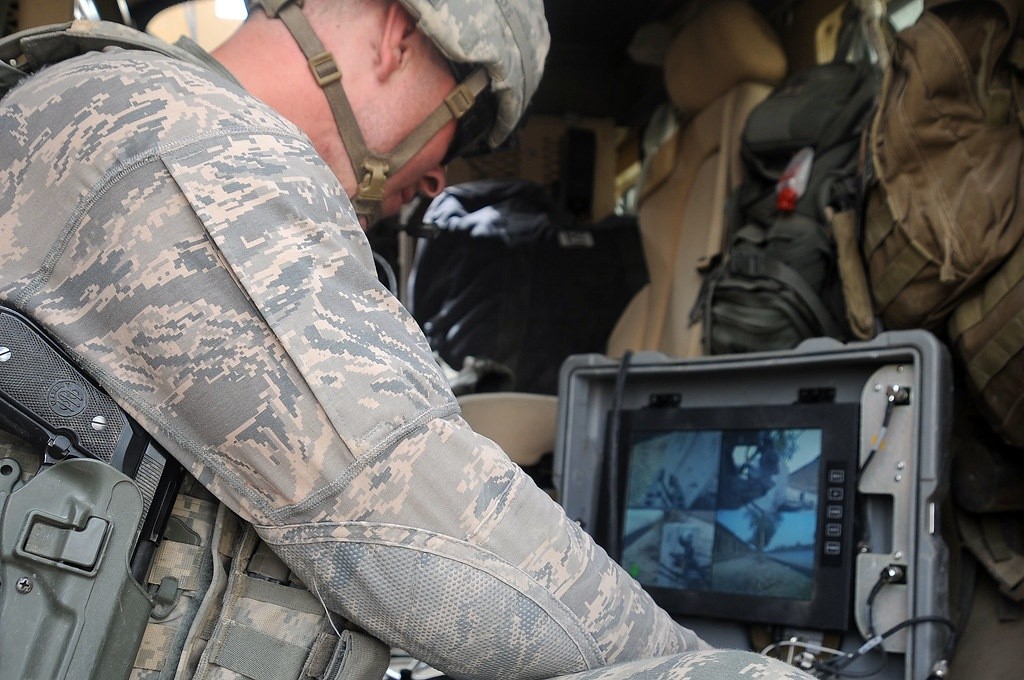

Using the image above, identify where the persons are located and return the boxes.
[0,0,822,680]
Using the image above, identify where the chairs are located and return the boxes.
[450,0,790,506]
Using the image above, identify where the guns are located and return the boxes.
[0,303,187,680]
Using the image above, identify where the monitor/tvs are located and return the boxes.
[601,403,857,632]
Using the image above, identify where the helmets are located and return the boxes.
[244,0,550,151]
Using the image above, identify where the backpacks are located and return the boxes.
[688,1,1022,602]
